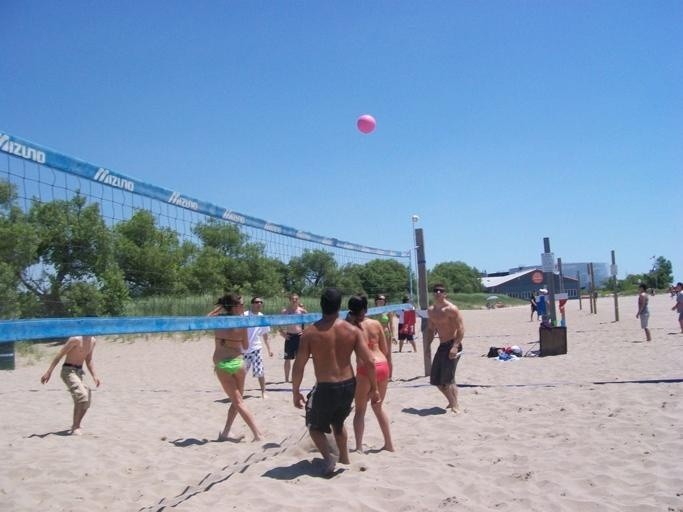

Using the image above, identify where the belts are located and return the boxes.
[62,362,82,369]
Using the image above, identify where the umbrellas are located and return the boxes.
[485,295,497,301]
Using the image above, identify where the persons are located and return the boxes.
[635,282,651,342]
[671,282,682,334]
[495,300,504,308]
[238,295,274,400]
[529,288,550,325]
[341,292,397,453]
[291,288,382,477]
[425,283,465,413]
[200,290,266,444]
[39,312,101,437]
[276,289,418,385]
[651,284,677,298]
[486,302,493,309]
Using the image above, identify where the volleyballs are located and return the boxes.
[357,115,375,133]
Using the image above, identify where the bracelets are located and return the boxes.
[452,343,459,348]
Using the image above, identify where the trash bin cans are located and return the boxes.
[0,342,15,370]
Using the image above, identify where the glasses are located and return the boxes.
[253,300,262,304]
[433,288,446,293]
